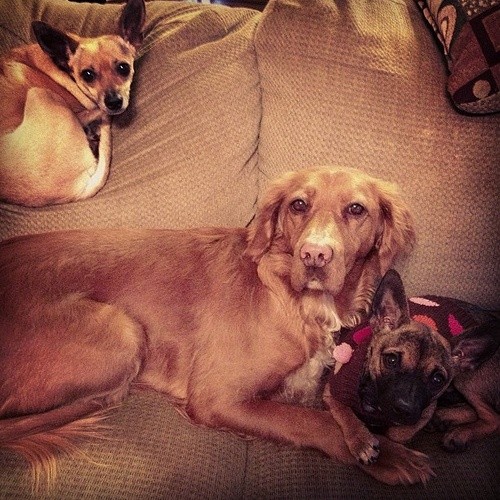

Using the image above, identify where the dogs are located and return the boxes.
[0,166,439,494]
[0,0,146,207]
[324,269,500,467]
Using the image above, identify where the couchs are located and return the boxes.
[0,0,500,500]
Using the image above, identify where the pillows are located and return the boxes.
[414,0,500,118]
